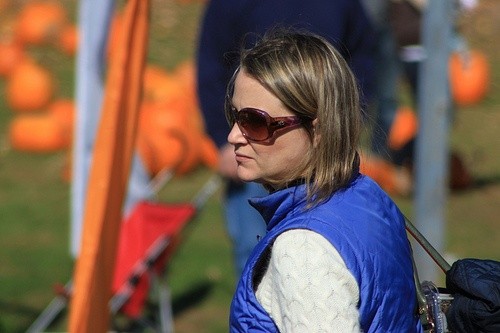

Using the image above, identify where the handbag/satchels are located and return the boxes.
[403,214,500,333]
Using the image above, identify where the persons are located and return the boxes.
[224,31,422,333]
[195,0,471,279]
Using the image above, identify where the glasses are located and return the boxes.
[223,97,308,143]
